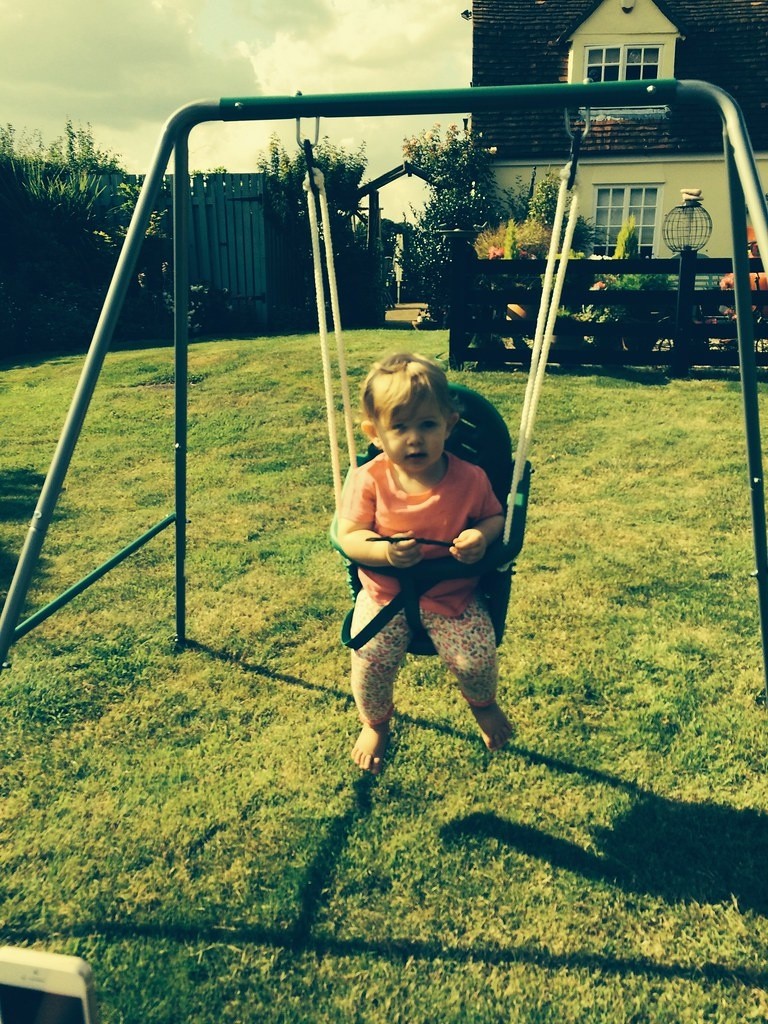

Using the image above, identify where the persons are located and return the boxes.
[335,349,512,777]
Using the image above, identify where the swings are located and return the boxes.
[301,130,582,657]
